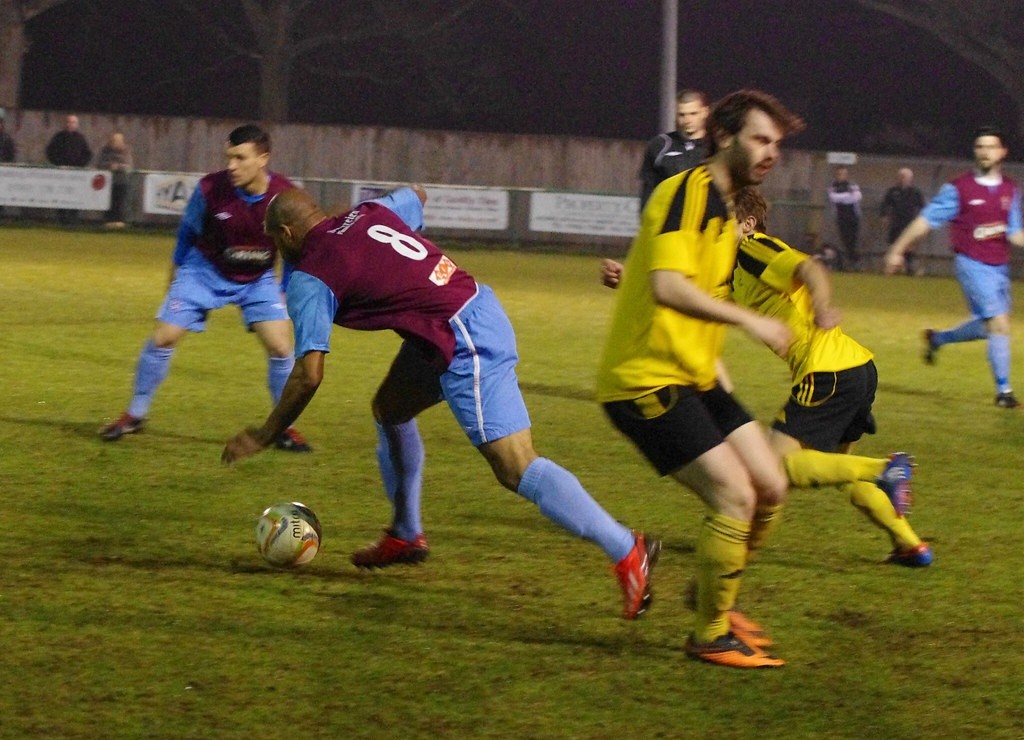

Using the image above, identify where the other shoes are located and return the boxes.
[922,328,941,365]
[994,392,1023,411]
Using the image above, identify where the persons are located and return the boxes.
[600,187,936,570]
[827,165,867,272]
[97,133,134,231]
[0,106,18,163]
[636,88,718,222]
[96,123,314,455]
[885,126,1024,412]
[879,167,926,276]
[45,113,95,231]
[600,88,808,672]
[222,185,665,623]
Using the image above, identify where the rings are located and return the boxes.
[601,265,607,272]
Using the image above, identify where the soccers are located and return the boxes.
[256,502,322,569]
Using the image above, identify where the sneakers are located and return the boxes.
[685,582,785,667]
[887,548,932,566]
[101,416,144,440]
[876,452,919,518]
[276,432,309,451]
[352,529,428,570]
[615,529,662,619]
